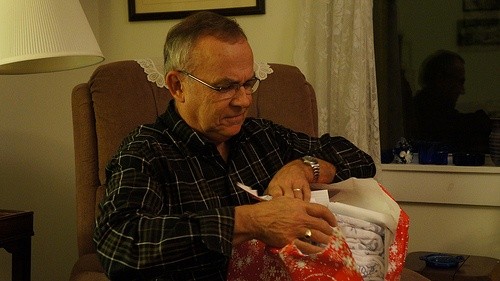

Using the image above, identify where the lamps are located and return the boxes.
[0,0,105,74]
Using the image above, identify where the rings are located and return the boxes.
[292,188,301,191]
[304,228,311,241]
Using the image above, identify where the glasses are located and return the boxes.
[177,70,261,95]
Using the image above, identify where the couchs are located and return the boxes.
[67,57,431,281]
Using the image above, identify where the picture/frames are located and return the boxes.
[128,0,265,21]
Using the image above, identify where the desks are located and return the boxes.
[0,208,35,281]
[405,251,500,281]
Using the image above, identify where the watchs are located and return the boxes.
[301,154,321,183]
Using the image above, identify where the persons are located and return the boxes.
[408,49,494,167]
[91,9,377,281]
